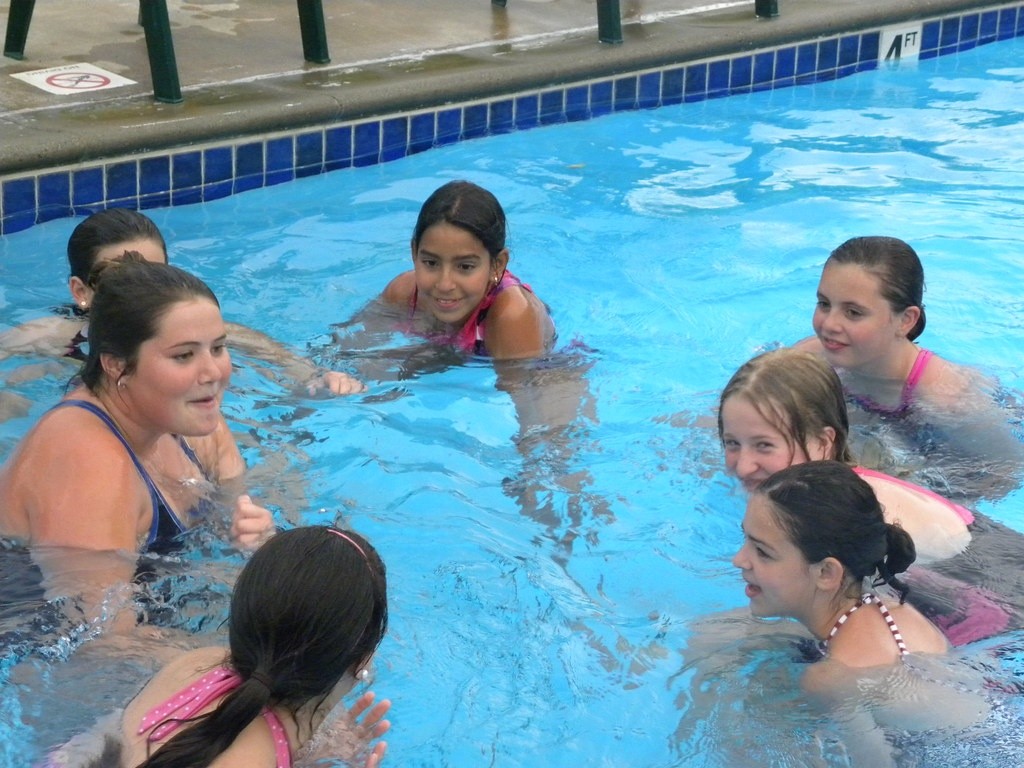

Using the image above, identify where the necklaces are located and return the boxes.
[154,450,194,487]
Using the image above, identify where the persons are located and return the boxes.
[716,235,1024,768]
[0,251,285,664]
[43,522,392,768]
[383,181,554,356]
[0,208,367,423]
